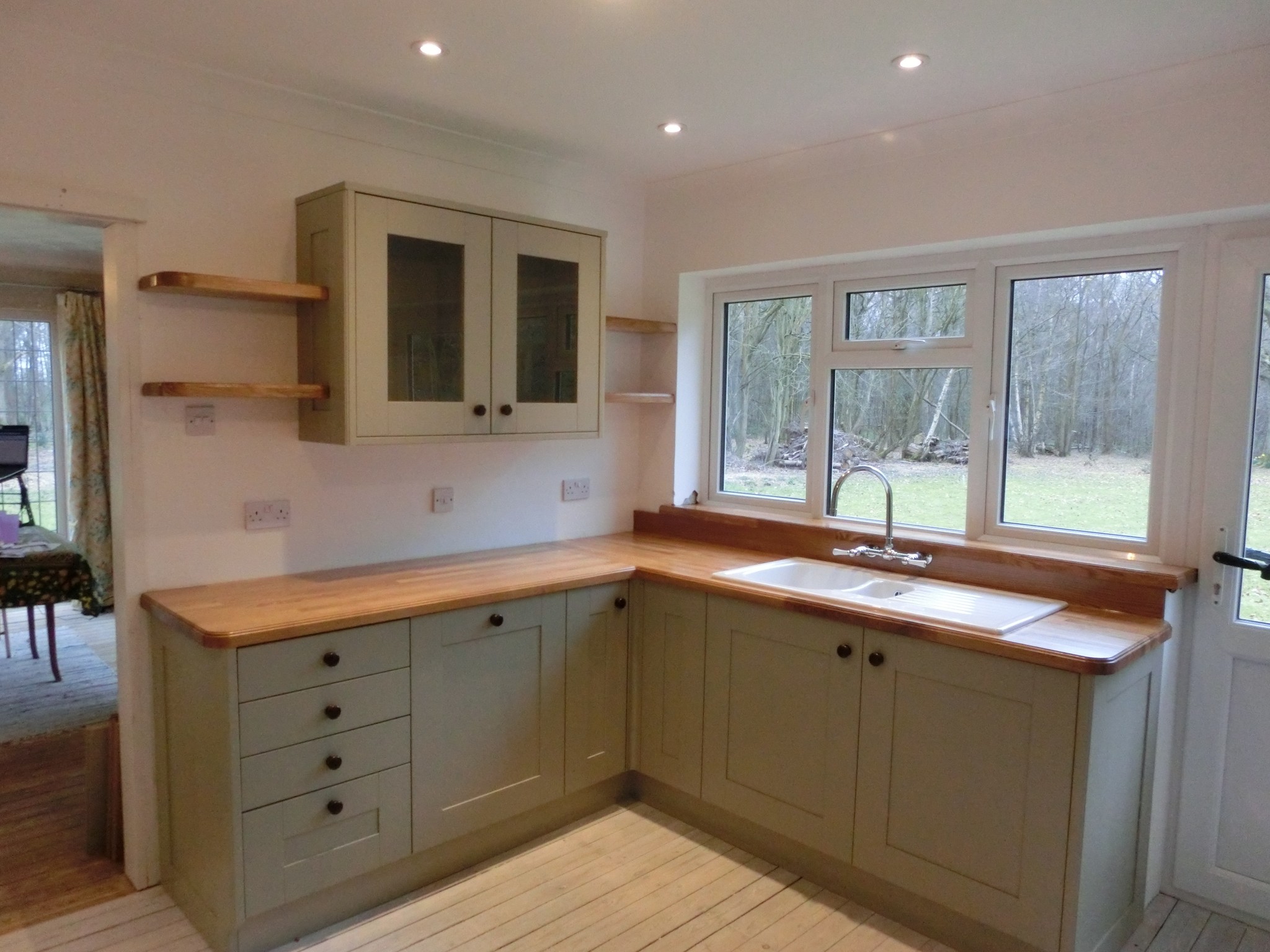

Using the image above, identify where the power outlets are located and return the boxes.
[560,476,591,502]
[242,497,292,532]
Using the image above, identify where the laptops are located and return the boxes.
[0,424,29,482]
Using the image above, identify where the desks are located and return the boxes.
[0,520,85,687]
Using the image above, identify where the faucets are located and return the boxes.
[829,464,933,569]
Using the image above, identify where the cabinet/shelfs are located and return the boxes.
[290,176,611,451]
[139,271,329,401]
[145,578,1172,952]
[605,315,677,404]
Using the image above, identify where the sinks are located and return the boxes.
[710,554,876,600]
[838,576,917,601]
